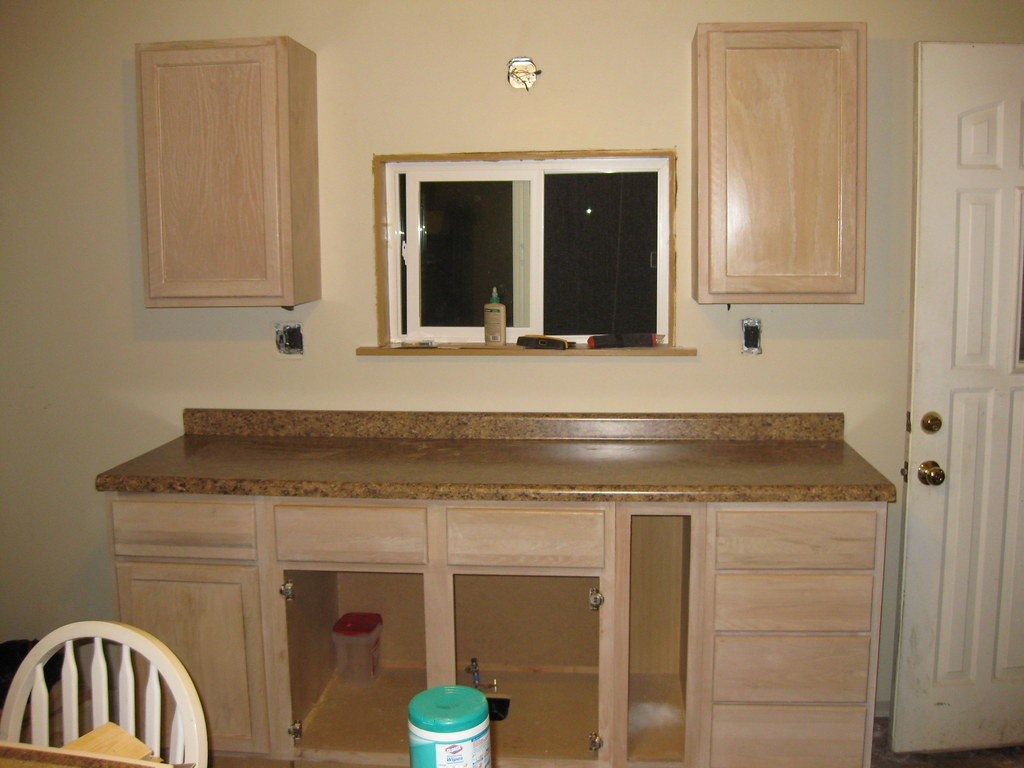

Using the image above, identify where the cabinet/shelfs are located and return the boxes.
[690,21,867,305]
[103,491,888,768]
[133,35,324,309]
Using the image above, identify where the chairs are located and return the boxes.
[0,621,208,768]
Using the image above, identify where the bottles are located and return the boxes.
[484,287,506,347]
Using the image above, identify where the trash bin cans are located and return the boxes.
[0,637,65,748]
